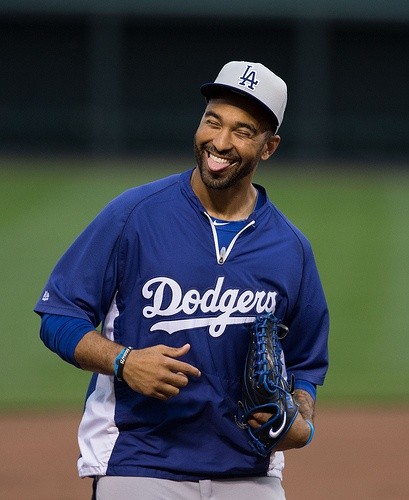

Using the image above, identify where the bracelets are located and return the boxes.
[114,346,134,383]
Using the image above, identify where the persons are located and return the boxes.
[34,62,331,500]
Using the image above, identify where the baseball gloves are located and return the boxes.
[236,313,300,460]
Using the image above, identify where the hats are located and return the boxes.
[201,59,289,135]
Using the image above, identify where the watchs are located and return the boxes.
[301,419,315,448]
